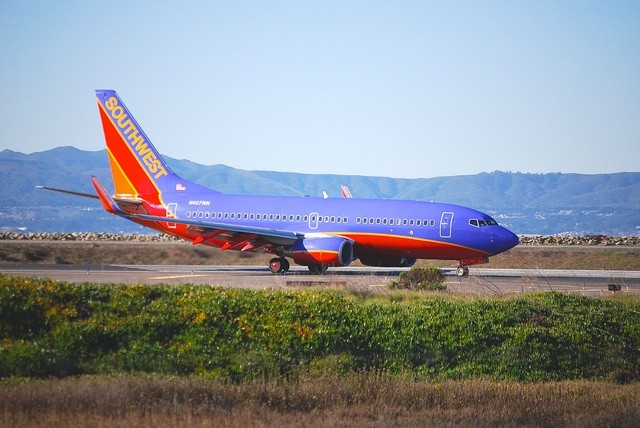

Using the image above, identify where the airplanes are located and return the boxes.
[34,89,519,276]
